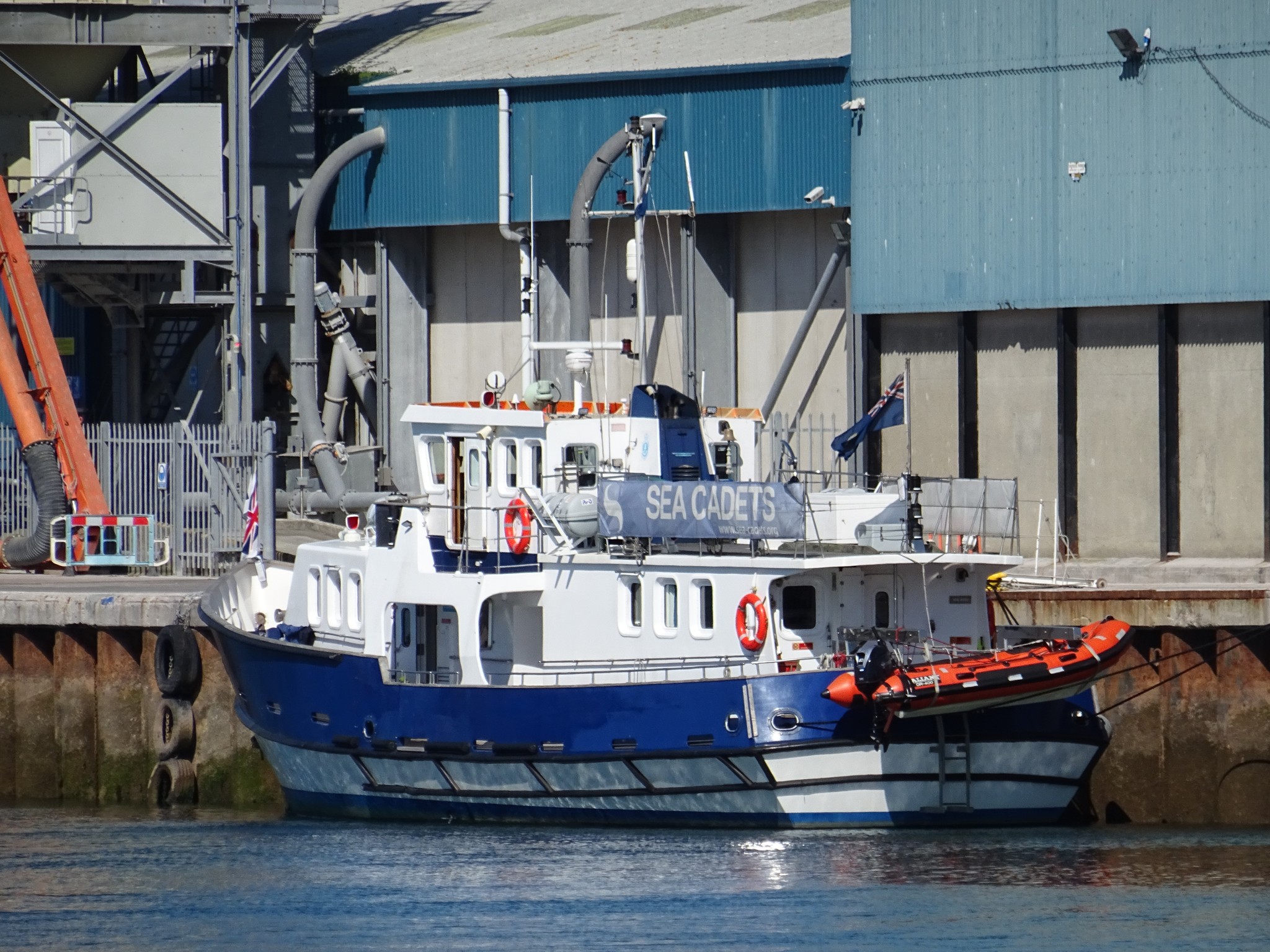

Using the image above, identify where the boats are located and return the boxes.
[820,615,1136,753]
[200,113,1112,830]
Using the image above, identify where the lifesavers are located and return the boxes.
[504,497,532,555]
[735,593,768,652]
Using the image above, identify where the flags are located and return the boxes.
[830,373,904,462]
[241,460,259,558]
